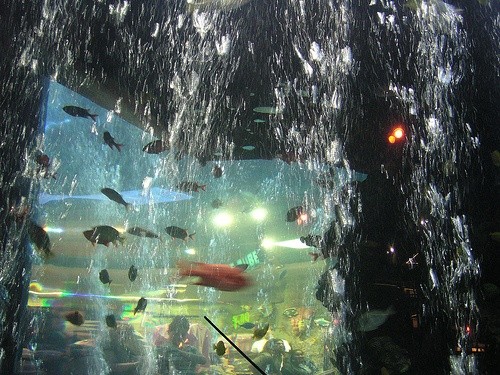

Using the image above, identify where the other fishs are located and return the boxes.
[239,322,255,329]
[279,267,288,280]
[82,229,120,248]
[165,225,196,243]
[141,139,171,154]
[175,180,207,194]
[127,264,139,283]
[104,314,118,330]
[127,226,163,243]
[212,165,224,179]
[27,221,55,258]
[62,105,99,122]
[299,234,322,249]
[351,303,397,333]
[212,341,226,357]
[133,297,148,317]
[208,198,225,209]
[250,322,269,341]
[92,225,127,246]
[35,152,59,181]
[99,269,113,287]
[282,307,300,318]
[284,205,305,223]
[59,309,84,327]
[103,131,124,153]
[100,187,132,212]
[313,317,332,327]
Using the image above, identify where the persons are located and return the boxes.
[26,291,210,375]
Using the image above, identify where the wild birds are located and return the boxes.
[174,257,257,292]
